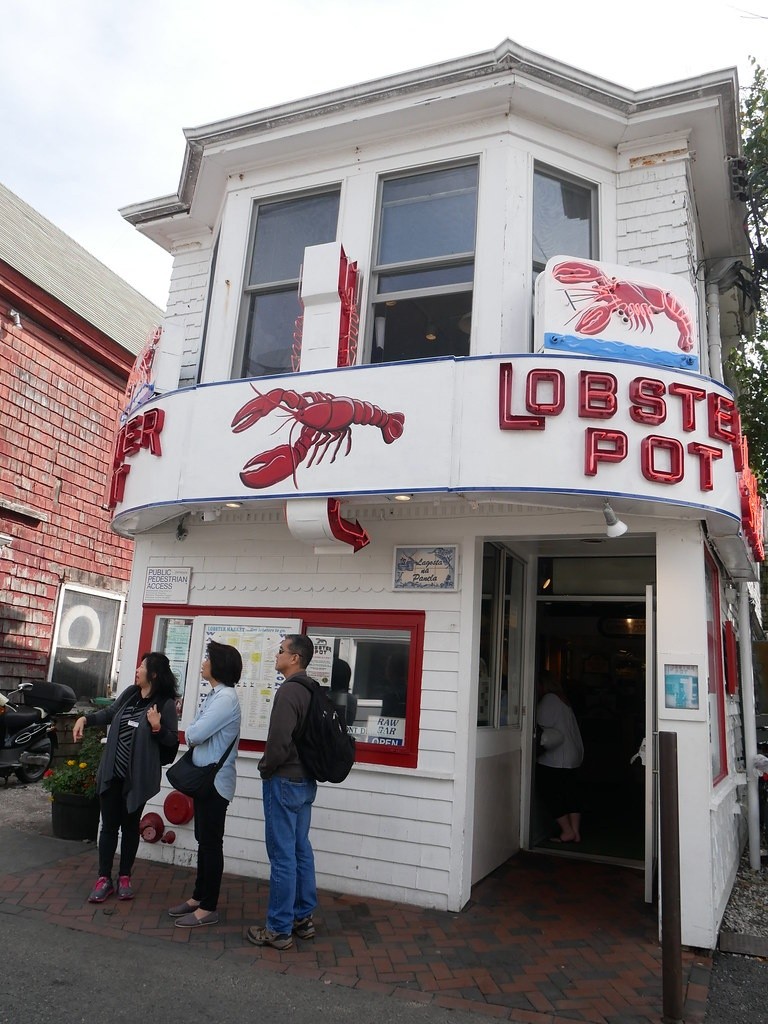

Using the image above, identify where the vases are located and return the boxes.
[51,792,99,840]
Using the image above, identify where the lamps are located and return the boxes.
[601,497,628,538]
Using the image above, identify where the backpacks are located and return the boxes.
[289,678,356,783]
[120,685,180,766]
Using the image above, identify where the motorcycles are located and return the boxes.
[0,680,78,785]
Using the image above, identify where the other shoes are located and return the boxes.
[168,898,218,928]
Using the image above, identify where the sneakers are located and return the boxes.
[116,875,136,900]
[247,925,293,950]
[294,914,316,940]
[88,875,115,903]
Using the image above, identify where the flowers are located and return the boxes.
[42,733,104,803]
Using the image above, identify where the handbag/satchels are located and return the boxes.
[167,746,217,799]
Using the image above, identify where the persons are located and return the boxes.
[246,633,324,951]
[71,651,178,902]
[167,639,243,928]
[536,667,585,844]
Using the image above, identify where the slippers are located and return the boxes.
[546,837,581,844]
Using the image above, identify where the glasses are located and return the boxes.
[279,647,304,658]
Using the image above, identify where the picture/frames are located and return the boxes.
[391,545,459,592]
